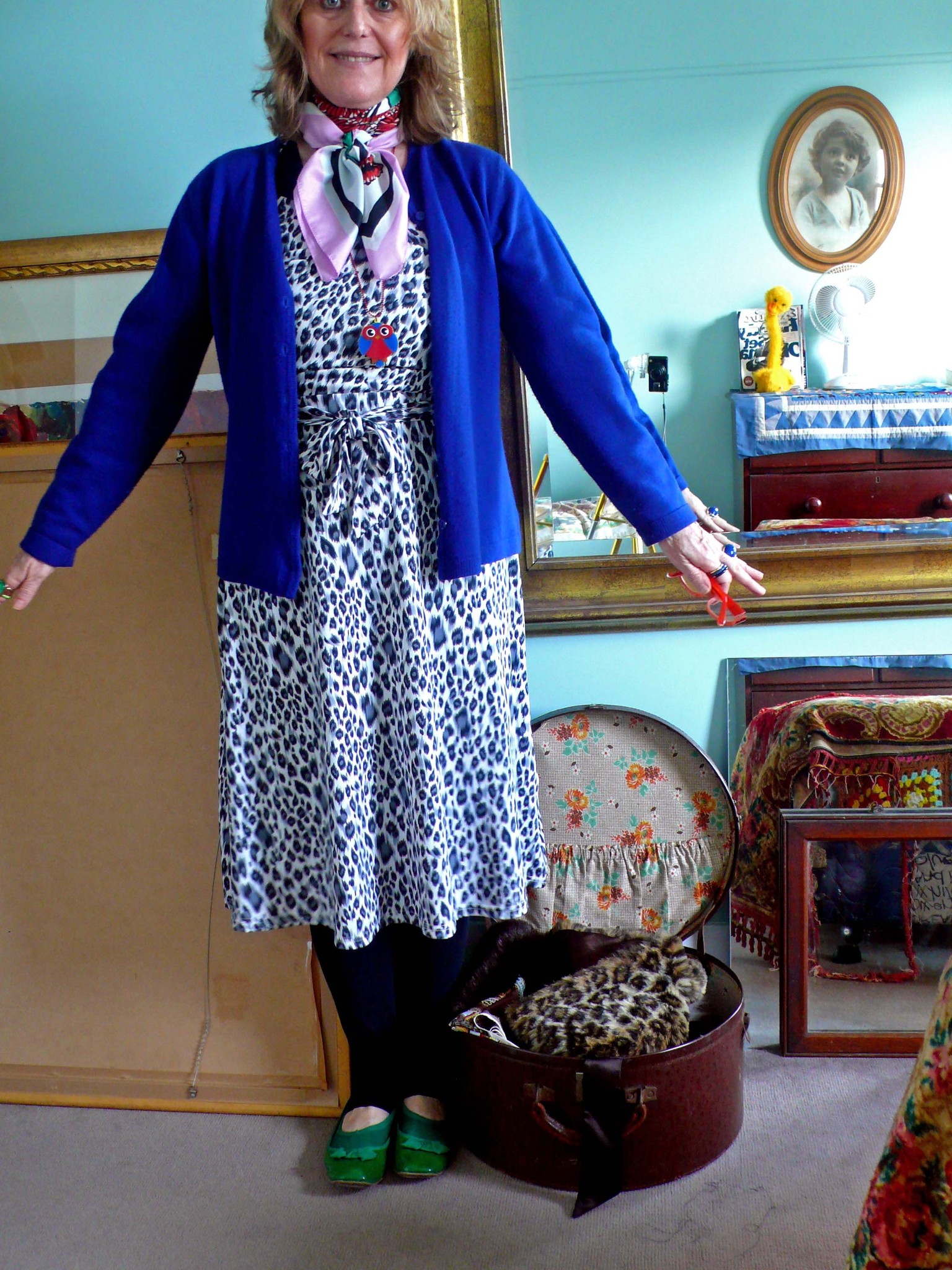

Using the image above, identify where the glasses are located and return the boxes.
[666,561,747,628]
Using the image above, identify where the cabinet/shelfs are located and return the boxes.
[730,390,948,522]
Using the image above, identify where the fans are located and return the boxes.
[809,262,884,391]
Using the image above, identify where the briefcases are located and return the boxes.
[436,704,750,1194]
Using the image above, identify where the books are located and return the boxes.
[737,305,808,391]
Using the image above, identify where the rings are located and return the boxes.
[722,544,737,557]
[707,506,719,518]
[710,563,729,578]
[0,580,13,599]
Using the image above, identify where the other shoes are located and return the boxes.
[392,1099,457,1177]
[324,1099,397,1188]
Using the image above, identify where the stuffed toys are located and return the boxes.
[752,286,795,392]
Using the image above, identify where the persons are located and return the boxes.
[746,321,785,372]
[794,120,871,253]
[0,0,767,1190]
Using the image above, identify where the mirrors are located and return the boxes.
[454,0,952,632]
[781,805,952,1056]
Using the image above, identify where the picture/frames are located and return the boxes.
[768,87,906,272]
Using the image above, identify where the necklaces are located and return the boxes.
[349,252,400,368]
